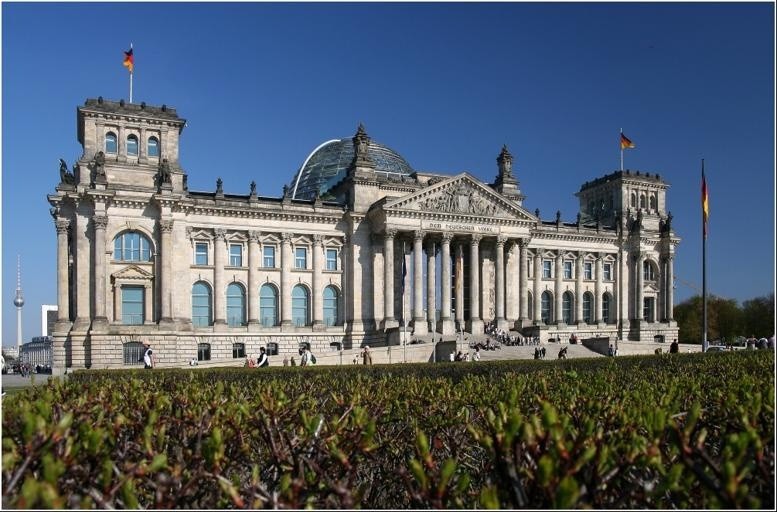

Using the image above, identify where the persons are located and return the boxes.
[9,362,50,379]
[669,339,678,353]
[189,358,198,366]
[418,184,497,216]
[95,150,105,176]
[158,159,171,182]
[299,343,312,367]
[253,346,268,369]
[360,345,373,366]
[558,346,569,360]
[608,344,619,356]
[142,341,155,369]
[557,334,561,344]
[637,208,642,220]
[2,354,6,396]
[446,321,546,363]
[687,348,691,353]
[667,211,672,222]
[745,334,775,350]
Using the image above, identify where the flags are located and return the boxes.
[121,48,134,73]
[620,132,635,150]
[700,175,709,241]
